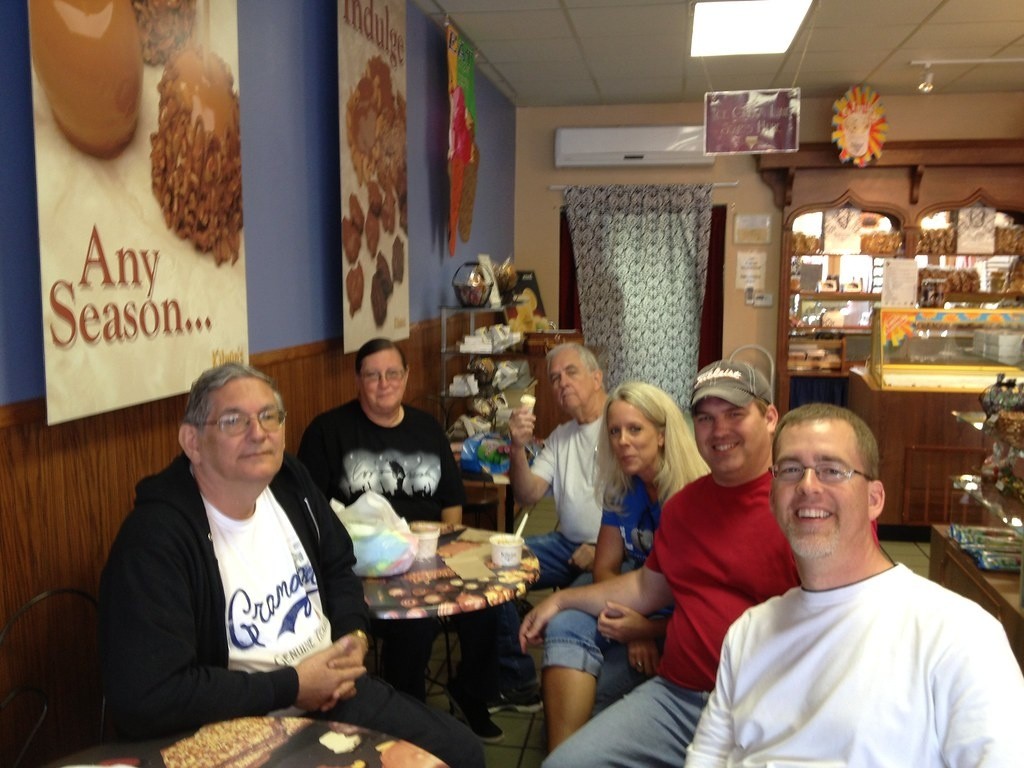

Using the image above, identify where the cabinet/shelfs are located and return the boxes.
[929,411,1024,676]
[913,186,1023,310]
[440,306,514,435]
[525,329,584,440]
[776,187,911,421]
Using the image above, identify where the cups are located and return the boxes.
[409,521,441,560]
[489,533,525,567]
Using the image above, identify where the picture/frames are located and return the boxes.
[502,268,546,323]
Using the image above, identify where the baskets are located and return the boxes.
[452,262,492,308]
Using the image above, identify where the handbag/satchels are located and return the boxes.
[523,321,583,354]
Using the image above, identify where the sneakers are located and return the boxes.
[487,684,543,711]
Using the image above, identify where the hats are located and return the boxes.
[689,359,772,407]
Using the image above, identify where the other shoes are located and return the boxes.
[448,673,504,743]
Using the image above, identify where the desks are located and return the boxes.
[47,716,451,768]
[361,525,540,715]
[454,454,506,532]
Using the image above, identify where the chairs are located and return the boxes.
[1,587,108,768]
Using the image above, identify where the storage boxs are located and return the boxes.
[972,329,1024,365]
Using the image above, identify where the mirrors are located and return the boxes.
[789,207,1024,335]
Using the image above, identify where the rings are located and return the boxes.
[635,662,644,667]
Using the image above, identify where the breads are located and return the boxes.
[794,224,1024,294]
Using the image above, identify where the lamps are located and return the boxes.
[917,73,934,93]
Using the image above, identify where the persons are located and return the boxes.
[101,362,489,768]
[519,360,881,768]
[544,382,712,755]
[487,342,606,713]
[685,404,1024,768]
[294,337,504,742]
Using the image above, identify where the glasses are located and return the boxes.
[768,462,875,485]
[193,407,288,435]
[360,367,405,383]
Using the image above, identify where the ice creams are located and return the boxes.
[520,394,536,414]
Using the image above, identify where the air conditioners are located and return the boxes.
[553,125,715,167]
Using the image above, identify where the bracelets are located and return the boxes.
[352,628,369,656]
